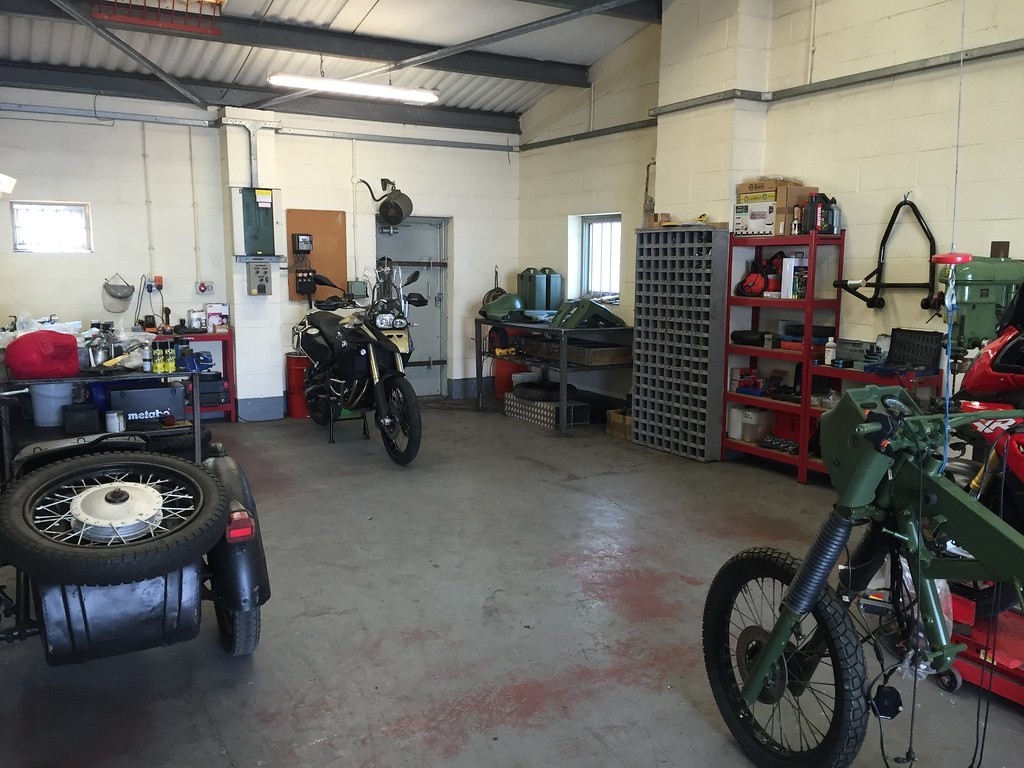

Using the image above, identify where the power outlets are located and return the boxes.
[155,276,162,290]
[196,281,215,294]
[145,282,154,291]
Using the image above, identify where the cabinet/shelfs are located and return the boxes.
[153,330,237,422]
[722,227,846,483]
[802,357,944,482]
[632,227,730,462]
[473,317,634,440]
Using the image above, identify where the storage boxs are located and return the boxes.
[605,409,633,442]
[734,179,819,236]
[187,302,230,333]
[63,403,101,435]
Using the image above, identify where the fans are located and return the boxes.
[379,179,414,226]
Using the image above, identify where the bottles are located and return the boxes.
[142,341,176,373]
[791,192,842,235]
[825,337,837,366]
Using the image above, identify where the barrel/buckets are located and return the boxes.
[511,372,540,389]
[285,352,313,418]
[29,383,75,428]
[495,359,529,401]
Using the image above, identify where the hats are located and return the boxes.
[102,273,134,313]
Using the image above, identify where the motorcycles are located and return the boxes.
[0,388,272,669]
[290,259,429,465]
[702,386,1023,768]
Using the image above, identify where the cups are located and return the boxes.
[105,410,127,433]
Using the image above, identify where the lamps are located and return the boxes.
[265,50,441,106]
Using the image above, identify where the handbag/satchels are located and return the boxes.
[5,329,78,378]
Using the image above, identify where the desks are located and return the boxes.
[0,370,202,481]
